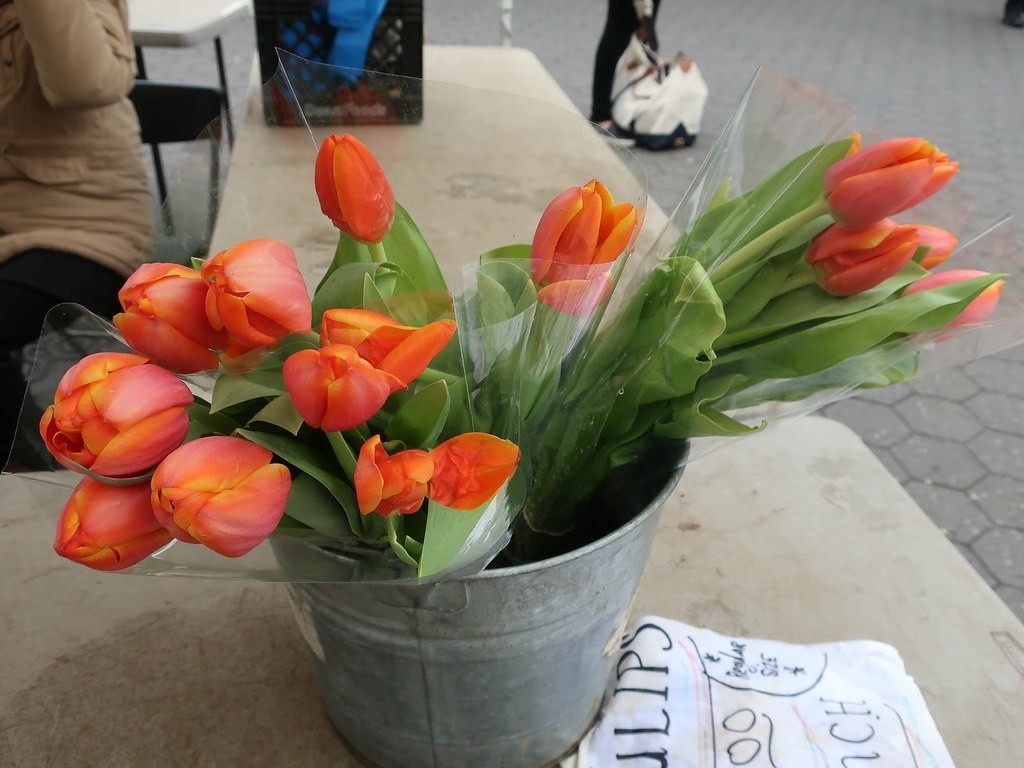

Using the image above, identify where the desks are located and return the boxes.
[0,45,1024,768]
[128,0,252,236]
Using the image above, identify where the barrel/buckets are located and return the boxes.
[267,438,690,768]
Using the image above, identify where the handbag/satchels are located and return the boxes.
[610,17,709,150]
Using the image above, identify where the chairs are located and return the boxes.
[126,81,225,267]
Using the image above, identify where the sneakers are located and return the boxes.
[592,123,636,147]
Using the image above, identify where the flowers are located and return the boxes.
[43,133,1010,586]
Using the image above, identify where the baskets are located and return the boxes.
[253,0,423,126]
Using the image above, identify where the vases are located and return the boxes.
[267,439,692,768]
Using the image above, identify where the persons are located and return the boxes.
[1001,0,1024,27]
[589,0,662,137]
[0,1,158,473]
